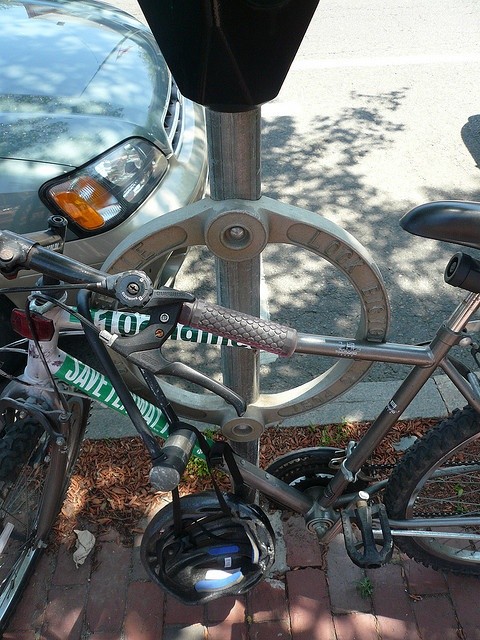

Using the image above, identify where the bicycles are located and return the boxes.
[0,200,480,640]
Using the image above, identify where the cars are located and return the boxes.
[0,0,210,389]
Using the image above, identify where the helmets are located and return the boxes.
[140,488,276,606]
